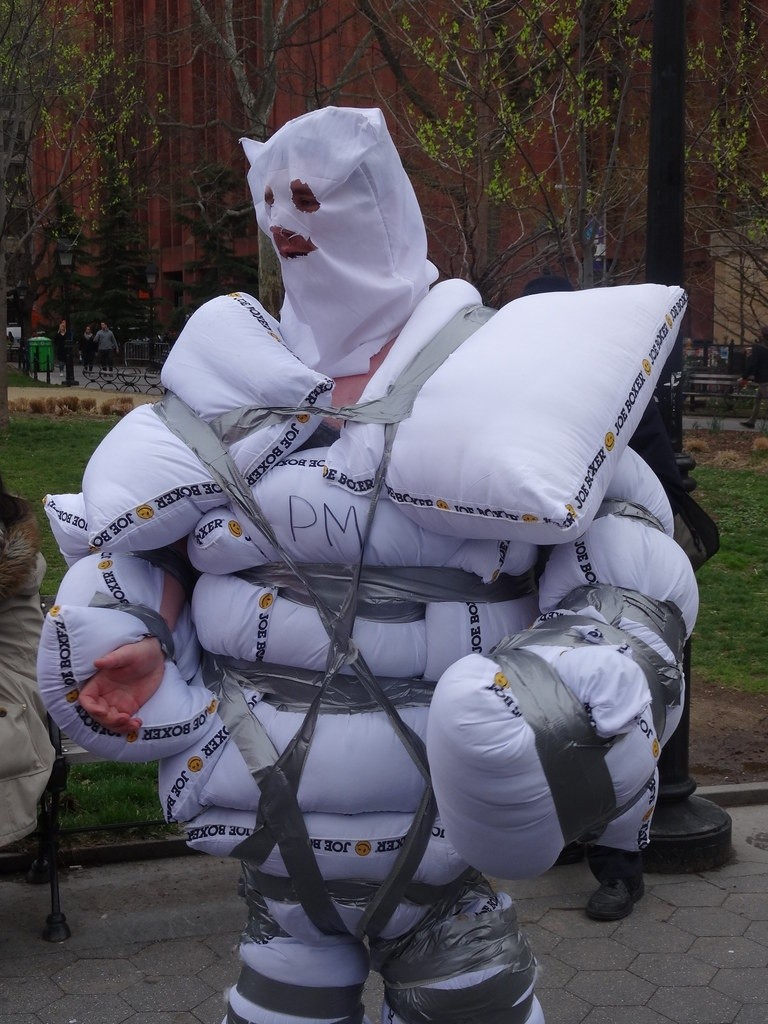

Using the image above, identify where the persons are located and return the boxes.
[522,274,688,924]
[38,104,707,1024]
[0,467,58,852]
[8,316,121,376]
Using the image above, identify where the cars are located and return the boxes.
[161,308,195,361]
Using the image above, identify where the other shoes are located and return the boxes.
[60,372,63,376]
[740,421,755,429]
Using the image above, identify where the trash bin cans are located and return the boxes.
[27,337,55,373]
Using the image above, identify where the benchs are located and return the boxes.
[41,710,134,944]
[681,373,768,413]
[83,364,167,396]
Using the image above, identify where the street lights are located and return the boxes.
[16,280,29,372]
[144,255,159,374]
[55,243,79,387]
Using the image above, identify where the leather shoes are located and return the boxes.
[586,877,644,922]
